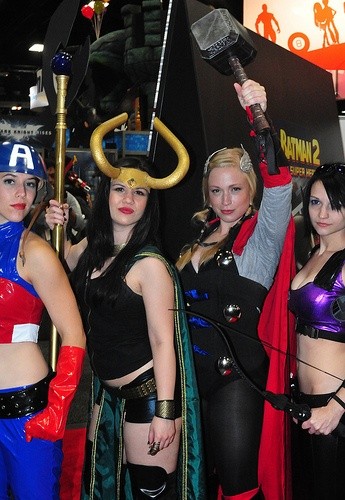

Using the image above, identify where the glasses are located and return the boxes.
[314,165,345,178]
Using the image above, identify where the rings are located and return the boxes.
[149,442,159,456]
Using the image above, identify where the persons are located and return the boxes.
[285,163,345,500]
[171,79,293,499]
[45,156,183,499]
[0,142,86,499]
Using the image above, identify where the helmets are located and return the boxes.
[0,140,47,181]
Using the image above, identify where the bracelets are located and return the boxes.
[156,401,175,420]
[330,392,344,409]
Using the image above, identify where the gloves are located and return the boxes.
[24,347,86,442]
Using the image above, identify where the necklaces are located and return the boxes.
[109,244,127,257]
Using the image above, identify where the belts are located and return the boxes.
[0,367,53,419]
[107,376,157,400]
